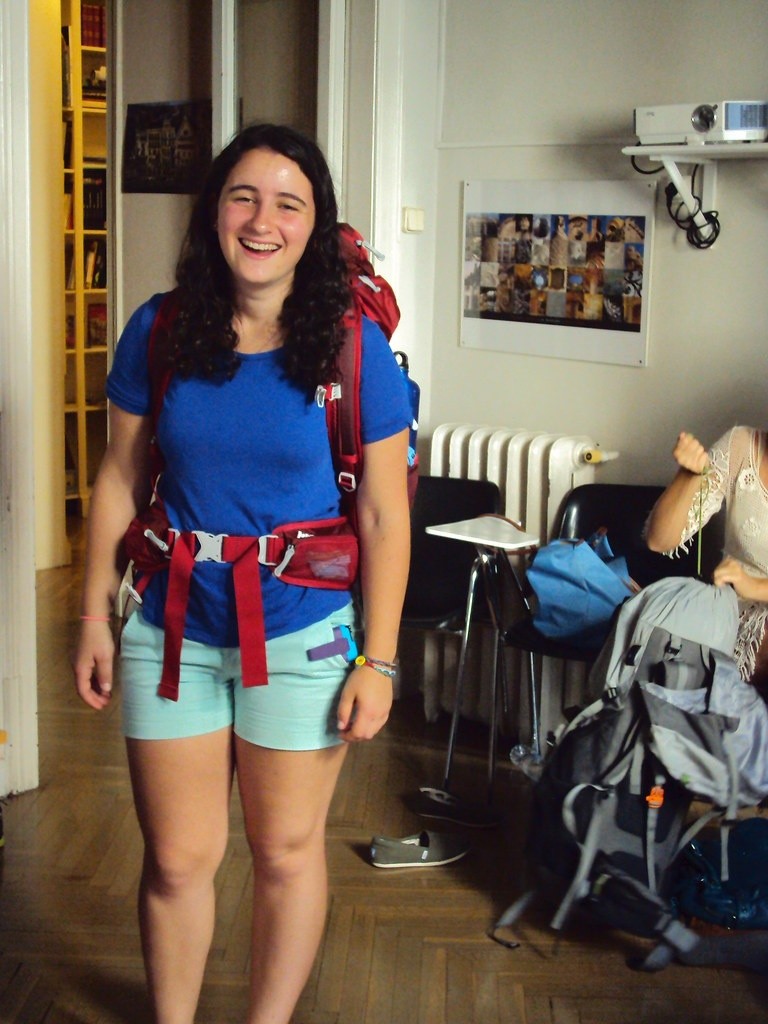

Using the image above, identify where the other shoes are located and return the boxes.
[369,828,473,869]
[410,783,505,828]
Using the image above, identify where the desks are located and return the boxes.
[424,514,541,807]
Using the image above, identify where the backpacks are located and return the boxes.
[114,220,419,703]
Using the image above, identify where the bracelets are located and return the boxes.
[364,656,396,677]
[79,616,110,621]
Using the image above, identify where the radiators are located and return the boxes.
[421,421,621,759]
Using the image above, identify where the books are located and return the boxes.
[64,176,107,349]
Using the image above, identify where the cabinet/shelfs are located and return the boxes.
[57,0,111,507]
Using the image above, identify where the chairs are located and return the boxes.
[399,476,708,785]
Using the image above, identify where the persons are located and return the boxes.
[641,426,768,682]
[74,123,413,1024]
